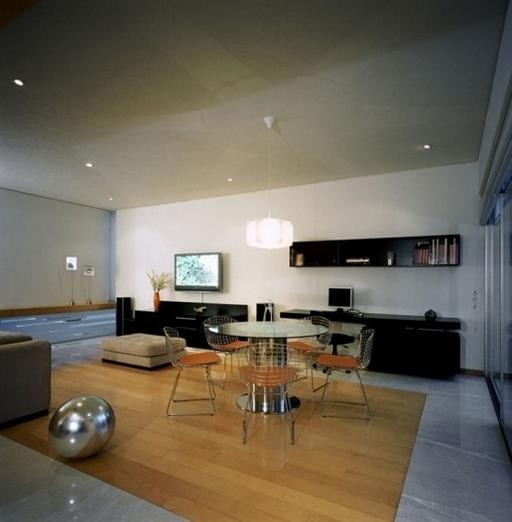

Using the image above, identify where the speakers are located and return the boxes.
[115,297,131,337]
[256,303,275,322]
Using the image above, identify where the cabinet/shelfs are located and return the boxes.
[280,309,462,381]
[289,233,460,267]
[134,300,248,351]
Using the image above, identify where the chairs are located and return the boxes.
[284,316,330,392]
[163,327,221,417]
[203,316,256,389]
[318,329,374,419]
[236,343,312,445]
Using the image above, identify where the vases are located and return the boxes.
[152,289,160,311]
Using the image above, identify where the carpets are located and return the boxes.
[0,346,427,522]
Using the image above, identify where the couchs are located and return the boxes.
[0,334,52,422]
[101,334,186,368]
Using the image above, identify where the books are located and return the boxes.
[413,236,456,265]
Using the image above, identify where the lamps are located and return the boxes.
[83,265,95,304]
[246,116,295,249]
[65,256,77,305]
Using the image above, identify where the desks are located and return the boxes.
[208,322,329,415]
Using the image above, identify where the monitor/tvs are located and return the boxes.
[174,252,223,291]
[328,288,353,312]
